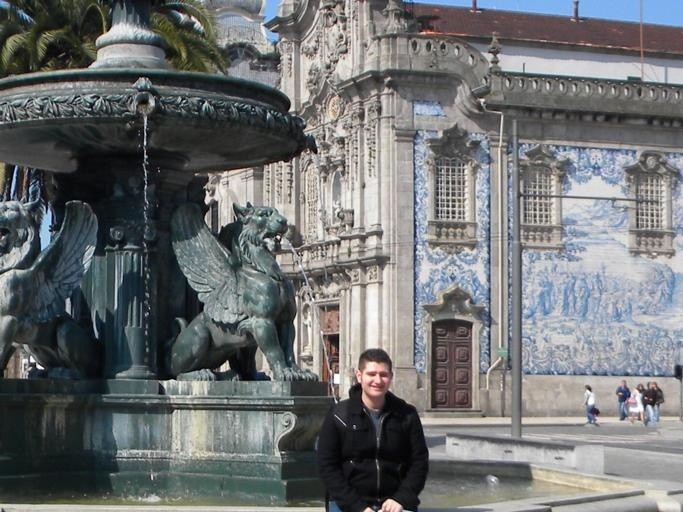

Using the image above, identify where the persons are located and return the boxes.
[651,383,665,423]
[616,380,631,421]
[315,348,430,511]
[628,383,646,426]
[640,381,656,427]
[581,384,598,427]
